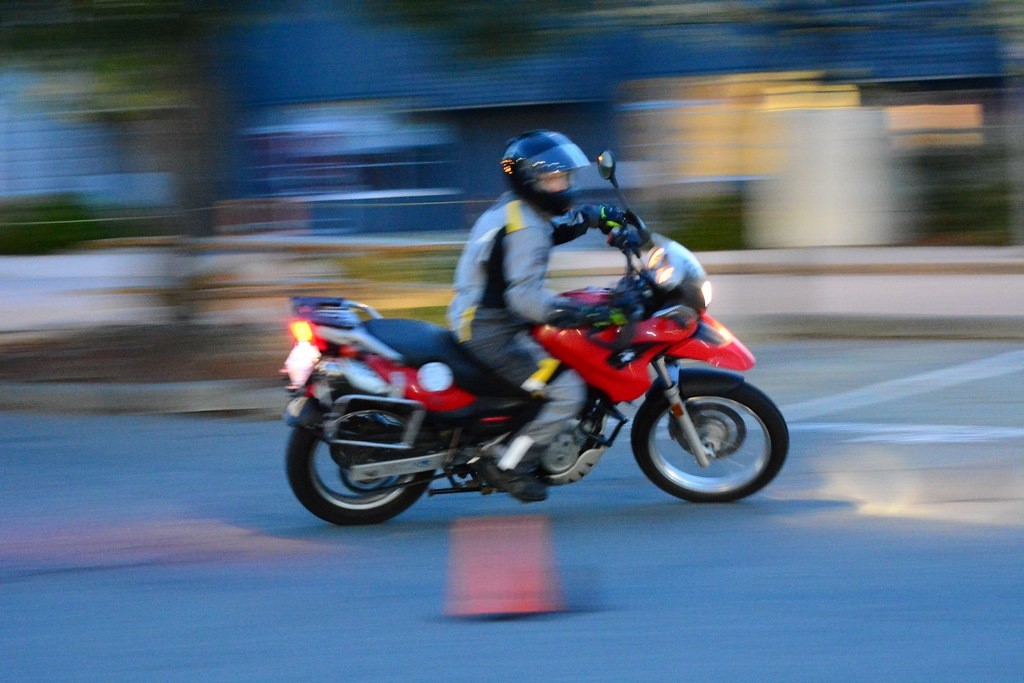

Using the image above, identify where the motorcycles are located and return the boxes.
[284,148,789,525]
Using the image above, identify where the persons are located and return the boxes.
[446,129,630,501]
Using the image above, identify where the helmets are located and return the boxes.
[501,127,590,217]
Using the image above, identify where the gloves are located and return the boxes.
[595,205,628,235]
[609,277,648,326]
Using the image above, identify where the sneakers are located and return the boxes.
[476,457,547,502]
